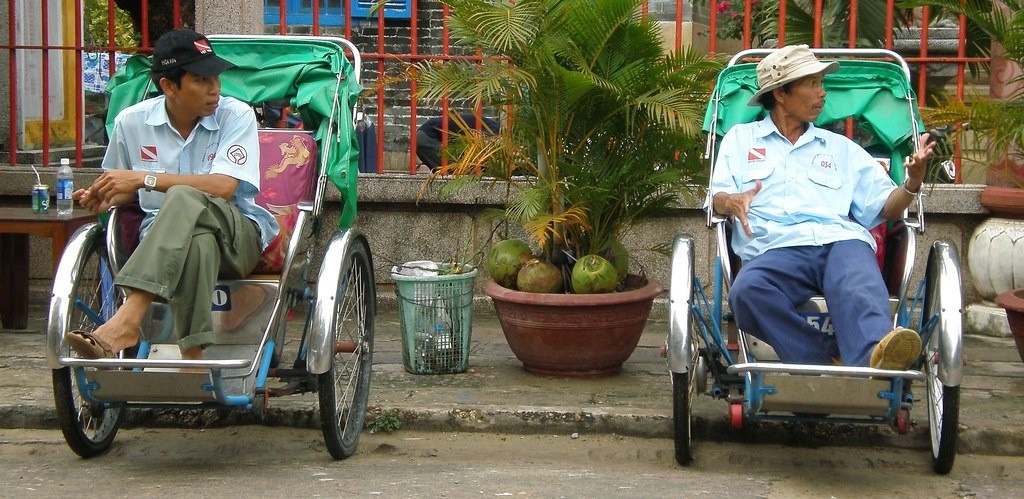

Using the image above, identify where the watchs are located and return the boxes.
[144,171,157,192]
[903,179,923,196]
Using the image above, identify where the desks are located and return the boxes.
[0,207,99,330]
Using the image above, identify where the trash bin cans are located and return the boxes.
[391,262,478,375]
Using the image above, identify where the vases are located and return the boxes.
[83,52,132,94]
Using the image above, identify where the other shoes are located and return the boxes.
[869,329,921,380]
[66,330,117,371]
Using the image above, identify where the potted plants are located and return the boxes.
[350,0,722,382]
[894,0,1024,362]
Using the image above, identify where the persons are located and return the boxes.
[704,45,937,370]
[67,29,279,374]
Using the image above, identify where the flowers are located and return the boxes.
[83,0,139,54]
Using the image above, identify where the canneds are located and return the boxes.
[32,185,50,213]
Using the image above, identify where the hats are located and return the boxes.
[747,44,839,106]
[152,29,237,76]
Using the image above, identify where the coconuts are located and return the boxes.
[488,238,533,289]
[571,254,618,294]
[517,257,564,294]
[609,243,630,286]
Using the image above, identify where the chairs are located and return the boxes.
[728,158,889,272]
[117,131,318,273]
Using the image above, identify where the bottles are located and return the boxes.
[56,158,74,215]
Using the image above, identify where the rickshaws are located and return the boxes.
[43,32,374,463]
[661,43,965,480]
